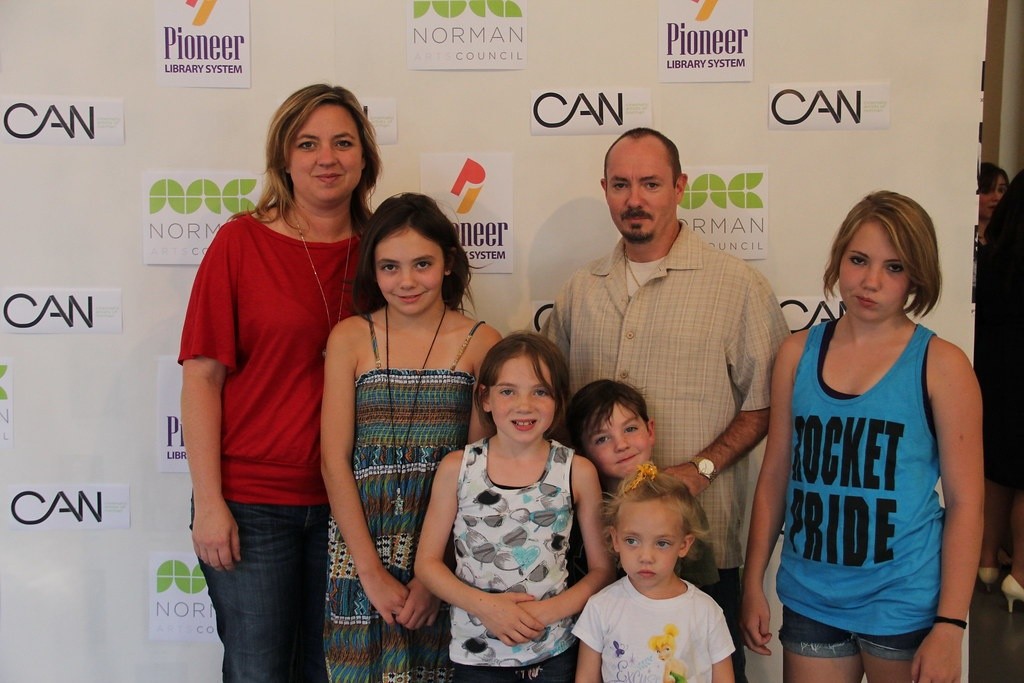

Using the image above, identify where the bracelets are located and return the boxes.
[933,613,968,629]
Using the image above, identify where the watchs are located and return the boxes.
[689,455,720,484]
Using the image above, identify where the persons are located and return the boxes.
[414,330,610,683]
[173,83,381,681]
[973,161,1009,300]
[538,126,792,682]
[317,190,500,681]
[569,472,740,683]
[973,165,1022,612]
[744,187,985,683]
[561,378,721,587]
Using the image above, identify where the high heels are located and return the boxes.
[980,567,1024,613]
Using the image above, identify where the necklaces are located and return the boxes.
[623,251,649,293]
[288,211,357,352]
[377,304,450,517]
